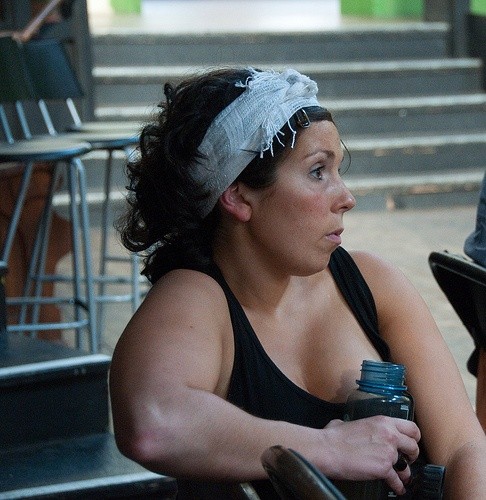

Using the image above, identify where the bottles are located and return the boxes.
[343,360,416,500]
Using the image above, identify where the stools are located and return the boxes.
[20,40,155,350]
[0,36,99,352]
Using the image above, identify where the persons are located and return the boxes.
[109,66,486,500]
[30,0,78,71]
